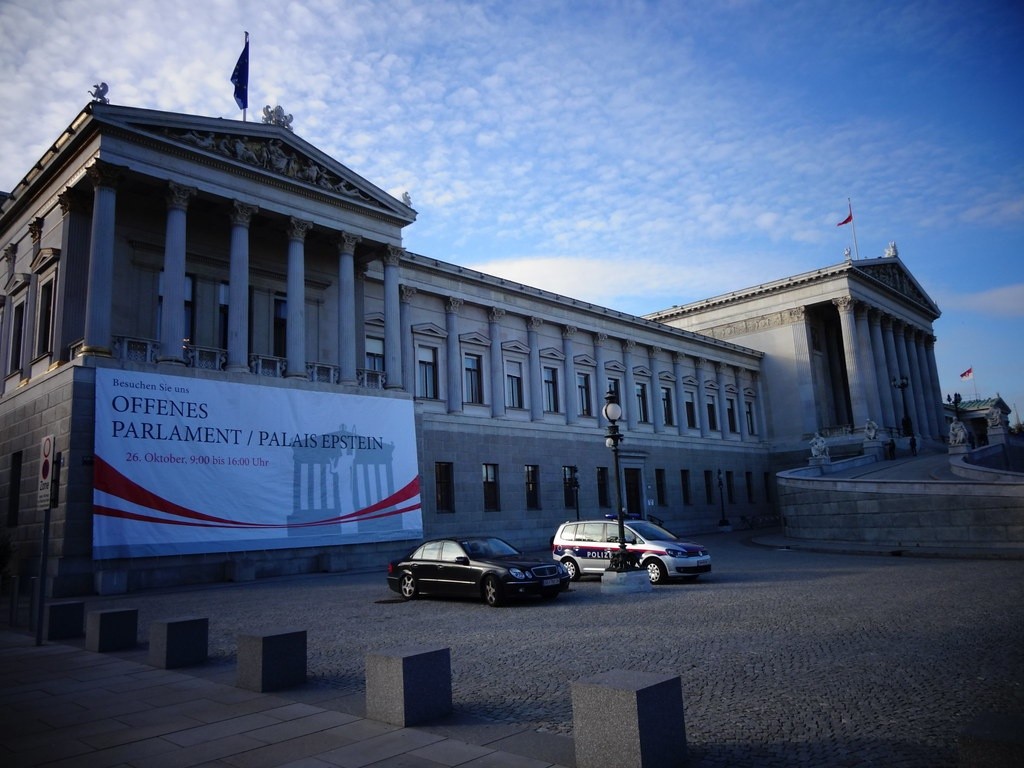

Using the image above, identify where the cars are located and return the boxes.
[387,539,571,608]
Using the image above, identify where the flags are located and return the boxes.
[960,368,974,382]
[837,203,852,227]
[230,32,249,110]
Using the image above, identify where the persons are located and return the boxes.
[885,439,897,460]
[864,419,878,441]
[984,402,1003,428]
[910,433,917,456]
[950,416,969,445]
[968,430,988,449]
[809,432,829,457]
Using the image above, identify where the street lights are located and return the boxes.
[601,384,630,573]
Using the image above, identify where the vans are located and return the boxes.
[551,513,712,585]
